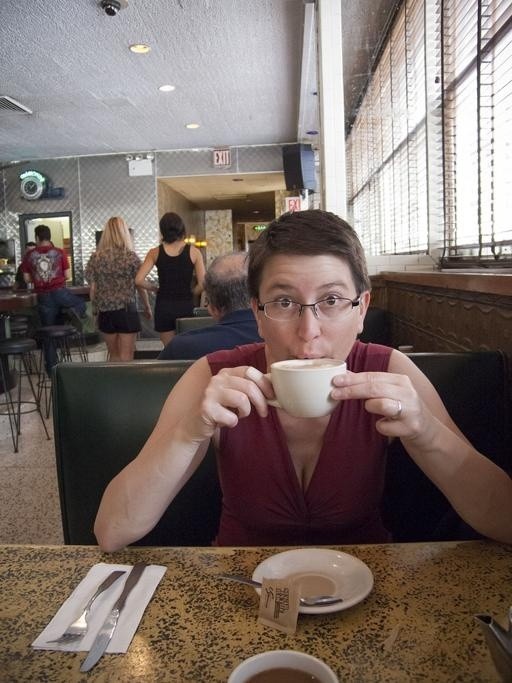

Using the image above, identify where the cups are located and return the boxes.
[226,648,338,682]
[1,257,9,265]
[257,358,347,418]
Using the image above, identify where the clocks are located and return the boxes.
[21,176,44,200]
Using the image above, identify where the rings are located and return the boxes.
[396,401,404,416]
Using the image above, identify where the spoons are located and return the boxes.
[212,570,343,607]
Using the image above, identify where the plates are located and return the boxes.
[252,547,376,614]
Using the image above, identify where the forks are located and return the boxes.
[48,568,127,647]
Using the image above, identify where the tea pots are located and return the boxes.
[474,602,512,682]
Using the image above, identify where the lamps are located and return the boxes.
[126,152,155,176]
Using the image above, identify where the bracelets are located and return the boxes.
[155,285,161,293]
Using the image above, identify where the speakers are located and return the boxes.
[282,143,317,191]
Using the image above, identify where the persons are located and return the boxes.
[21,223,88,379]
[137,210,207,348]
[152,251,266,360]
[81,216,150,362]
[90,210,511,548]
[14,241,35,288]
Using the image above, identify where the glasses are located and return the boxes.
[256,293,361,322]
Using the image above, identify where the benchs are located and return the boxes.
[50,351,508,545]
[176,305,392,347]
[194,306,212,315]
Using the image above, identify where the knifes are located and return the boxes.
[81,560,150,672]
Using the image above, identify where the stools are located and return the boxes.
[0,300,90,454]
